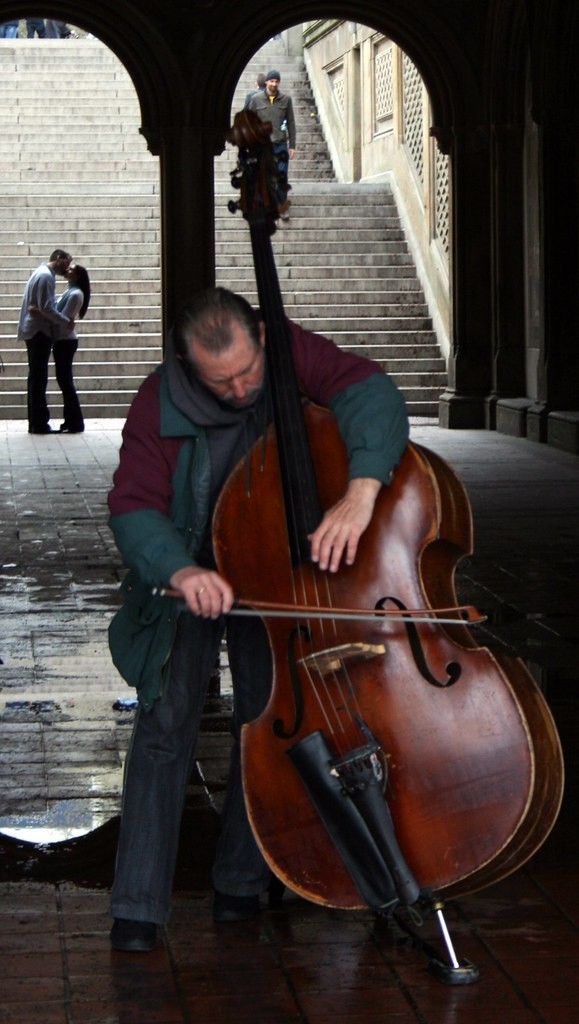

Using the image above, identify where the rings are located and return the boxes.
[197,585,204,593]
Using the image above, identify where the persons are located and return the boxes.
[18,250,91,434]
[245,70,296,220]
[0,18,100,39]
[106,291,406,951]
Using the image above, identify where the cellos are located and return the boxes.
[209,107,568,979]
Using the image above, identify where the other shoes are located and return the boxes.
[29,429,61,435]
[60,422,85,433]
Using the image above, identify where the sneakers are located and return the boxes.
[279,212,290,220]
[212,888,261,922]
[110,917,158,951]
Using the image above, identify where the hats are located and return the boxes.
[265,70,280,82]
